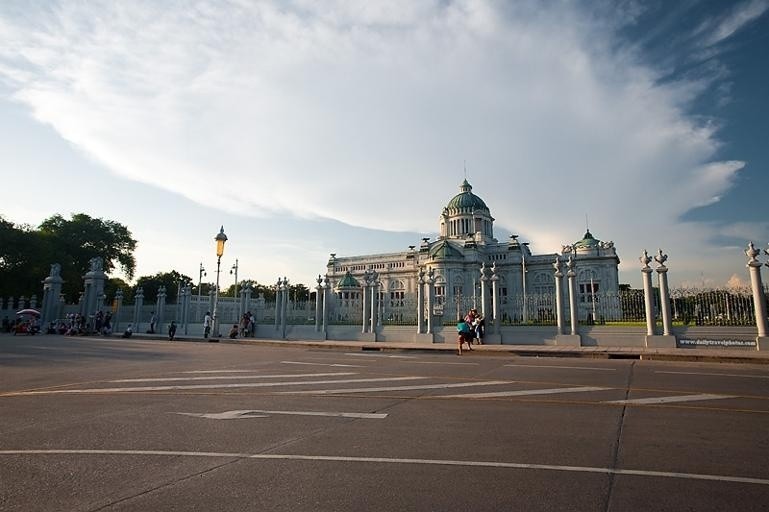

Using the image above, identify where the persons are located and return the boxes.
[229,324,238,339]
[456,318,473,356]
[464,311,474,346]
[203,312,212,339]
[239,311,255,337]
[1,305,114,337]
[122,324,133,338]
[471,308,486,345]
[168,320,177,341]
[150,311,156,333]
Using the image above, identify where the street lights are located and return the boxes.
[210,225,228,338]
